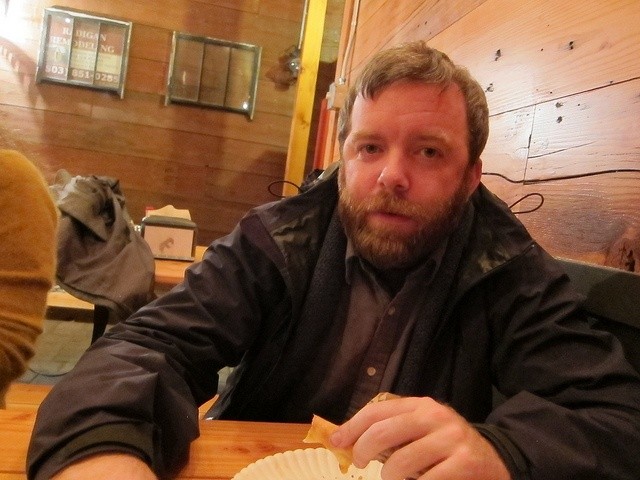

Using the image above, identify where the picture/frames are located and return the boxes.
[32,5,140,101]
[158,30,266,121]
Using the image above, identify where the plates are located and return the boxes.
[230,447,405,480]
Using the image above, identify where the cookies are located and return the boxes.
[303,414,360,475]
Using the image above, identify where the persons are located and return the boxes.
[0,148,59,410]
[26,41,640,480]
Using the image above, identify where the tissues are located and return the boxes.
[139,204,197,263]
